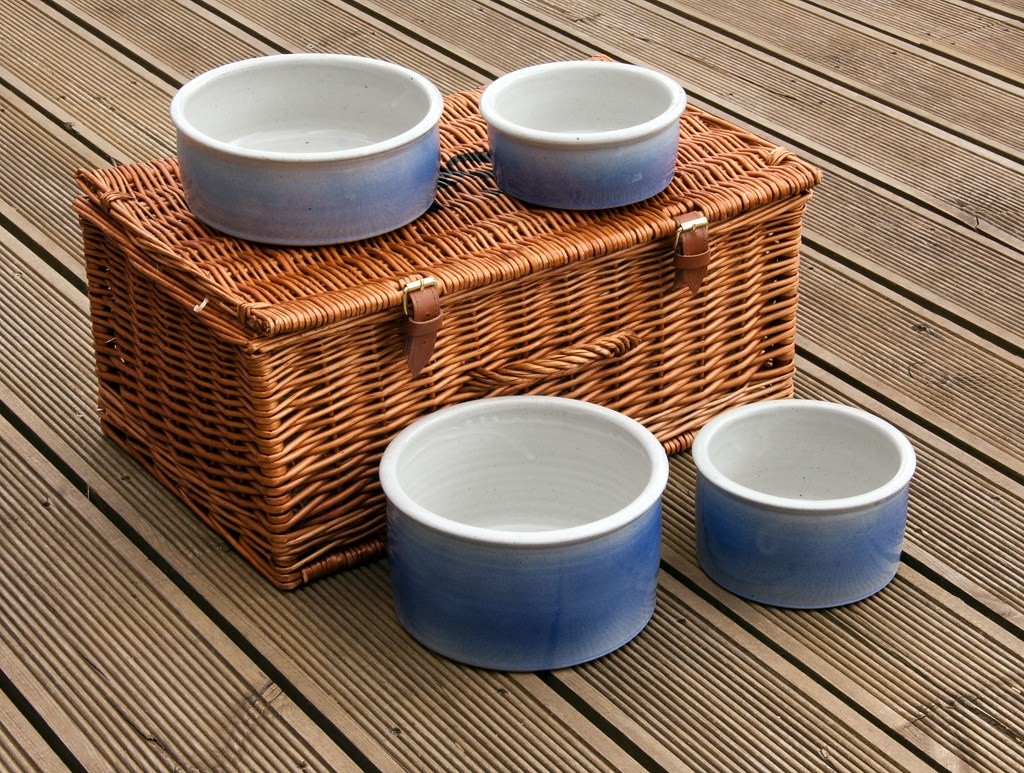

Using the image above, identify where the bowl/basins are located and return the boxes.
[478,62,687,211]
[168,53,444,247]
[377,397,667,674]
[690,394,915,610]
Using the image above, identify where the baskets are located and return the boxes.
[73,54,823,591]
